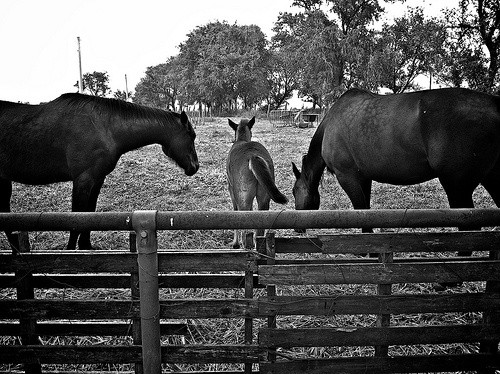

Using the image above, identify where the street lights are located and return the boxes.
[76,37,84,93]
[125,74,129,102]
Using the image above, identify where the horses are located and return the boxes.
[0,92,199,250]
[292,87,500,287]
[226,116,289,249]
